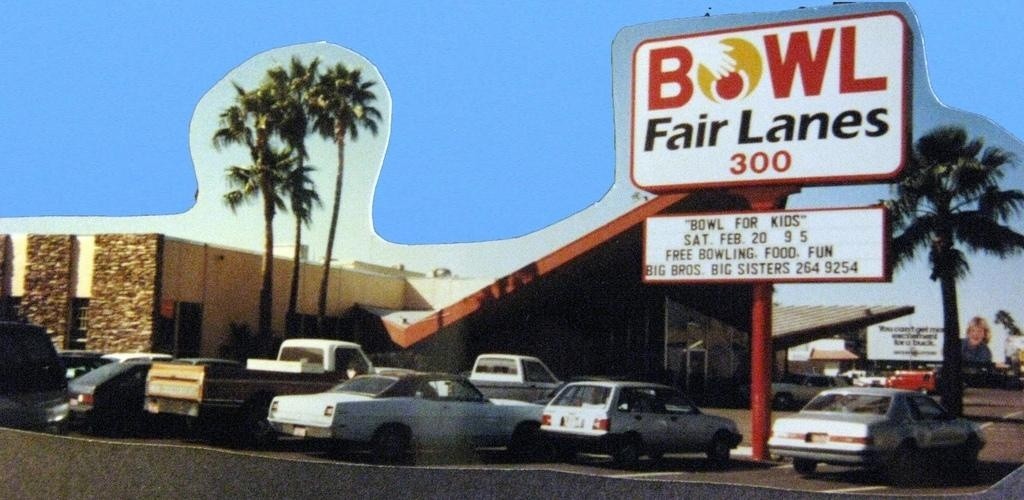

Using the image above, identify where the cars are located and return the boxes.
[534,378,741,461]
[269,372,546,463]
[0,320,247,436]
[738,372,985,485]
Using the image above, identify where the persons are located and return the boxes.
[959,316,993,374]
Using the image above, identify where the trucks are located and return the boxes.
[472,354,564,388]
[141,337,374,451]
